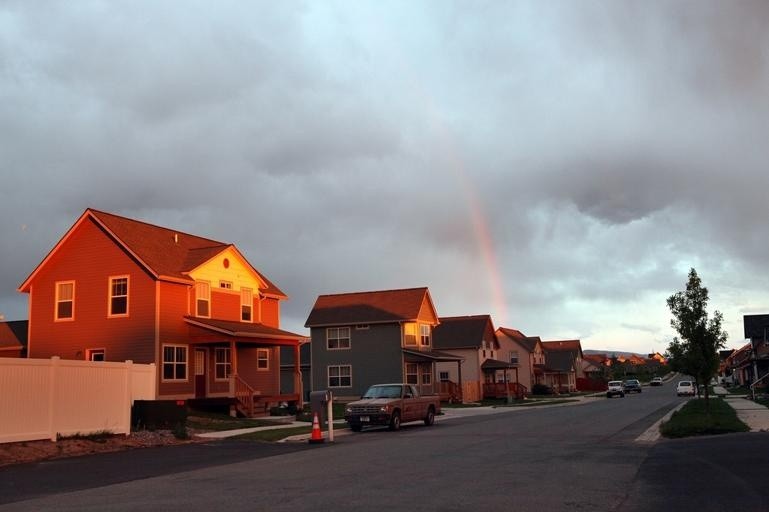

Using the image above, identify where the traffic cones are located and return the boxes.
[309,411,325,444]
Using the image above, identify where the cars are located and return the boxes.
[650,377,663,386]
[607,379,642,397]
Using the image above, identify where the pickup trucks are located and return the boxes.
[344,383,441,431]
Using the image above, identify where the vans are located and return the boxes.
[677,381,697,396]
